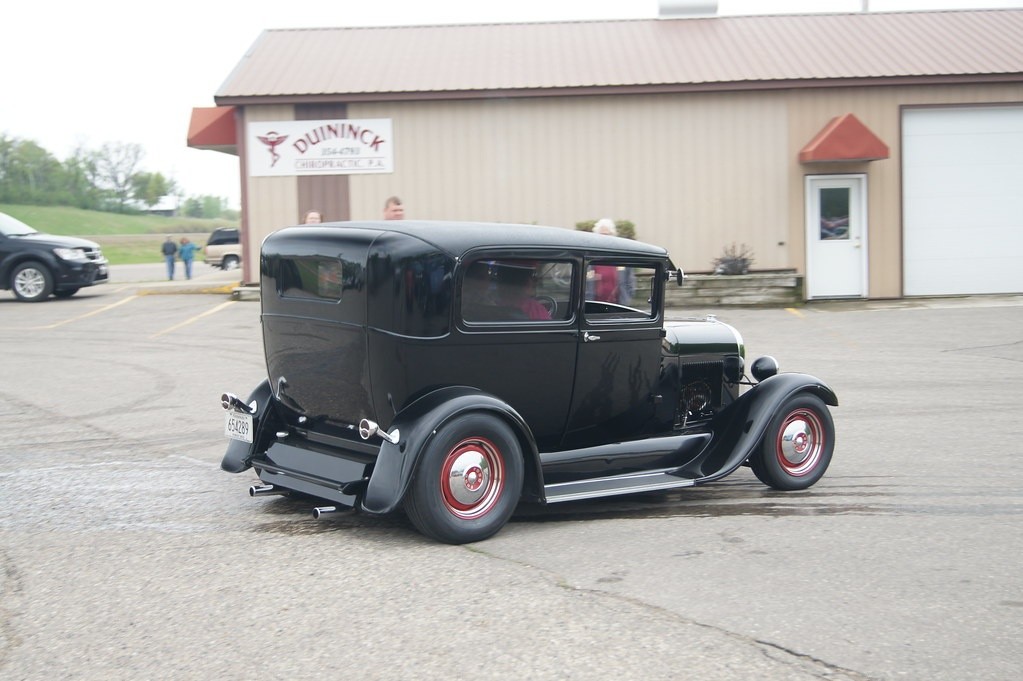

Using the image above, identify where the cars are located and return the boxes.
[220,222,840,543]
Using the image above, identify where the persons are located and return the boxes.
[383,196,404,220]
[302,209,323,224]
[496,259,552,320]
[178,237,202,280]
[576,219,635,307]
[162,235,176,280]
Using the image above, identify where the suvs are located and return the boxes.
[0,212,110,303]
[202,228,243,272]
